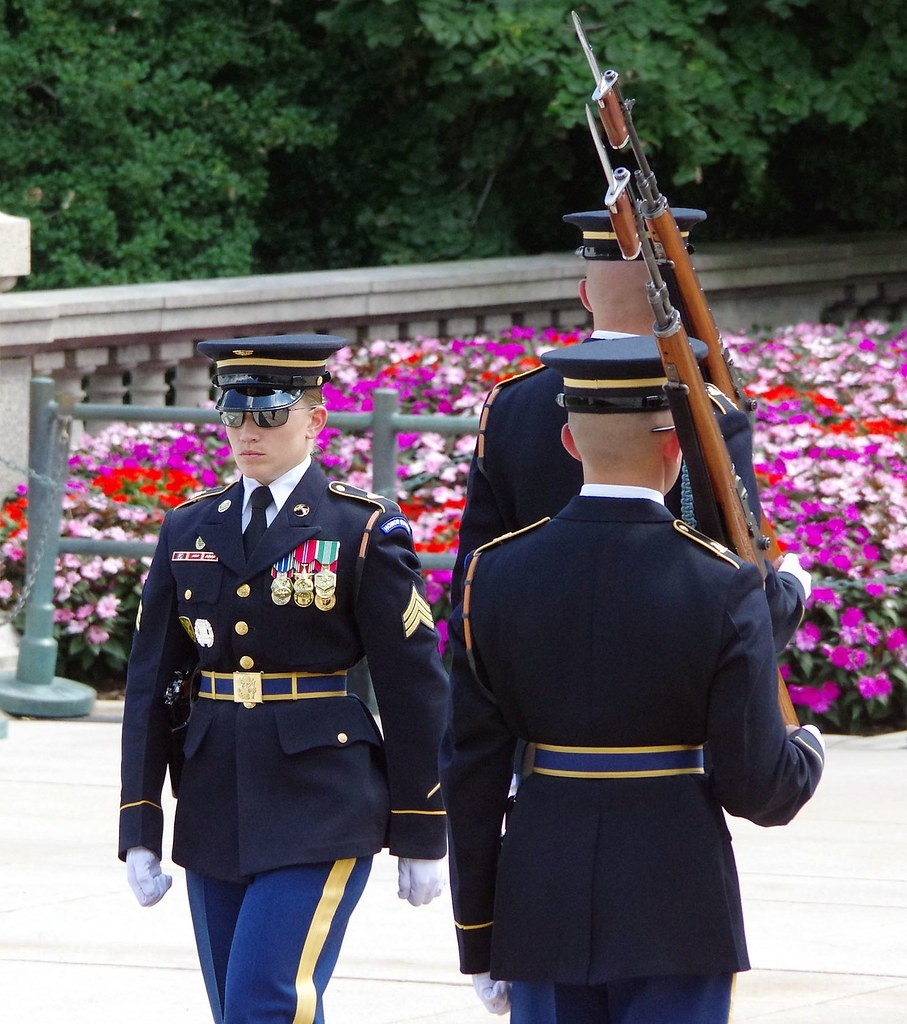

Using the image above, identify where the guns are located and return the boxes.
[581,99,801,740]
[567,9,782,561]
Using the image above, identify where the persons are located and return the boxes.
[435,209,825,1024]
[118,335,452,1024]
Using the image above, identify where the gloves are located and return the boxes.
[125,845,173,908]
[472,971,512,1016]
[777,552,812,600]
[397,856,443,907]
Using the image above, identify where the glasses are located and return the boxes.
[220,403,324,428]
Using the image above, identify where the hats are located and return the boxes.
[562,206,708,262]
[540,334,709,414]
[196,333,346,412]
